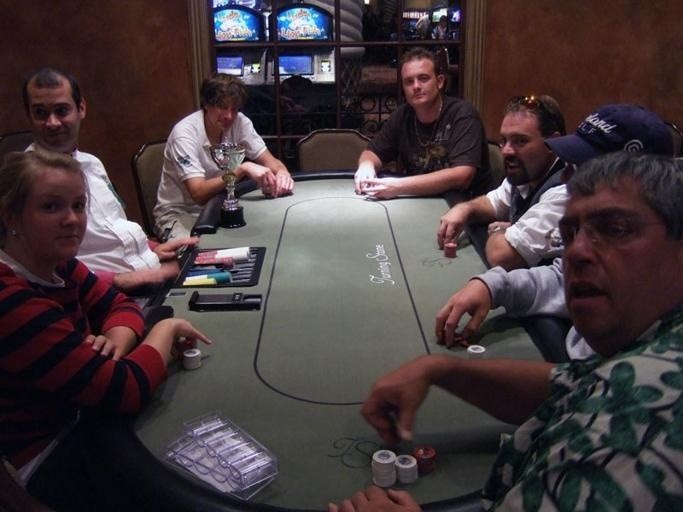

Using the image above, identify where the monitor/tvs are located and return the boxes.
[272,52,314,77]
[216,53,244,77]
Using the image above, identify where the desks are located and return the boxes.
[125,171,572,511]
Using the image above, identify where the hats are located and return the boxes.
[543,102,676,166]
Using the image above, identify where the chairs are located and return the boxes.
[483,140,507,188]
[130,140,167,233]
[293,128,371,173]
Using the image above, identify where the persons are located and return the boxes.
[431,16,454,41]
[435,95,569,274]
[0,140,212,512]
[415,10,433,40]
[354,50,487,265]
[326,154,683,512]
[435,103,683,362]
[153,73,294,264]
[24,63,200,312]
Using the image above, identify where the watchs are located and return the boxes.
[486,222,506,237]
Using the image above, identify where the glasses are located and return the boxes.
[509,94,543,110]
[559,208,661,245]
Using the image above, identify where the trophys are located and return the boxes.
[204,137,254,230]
